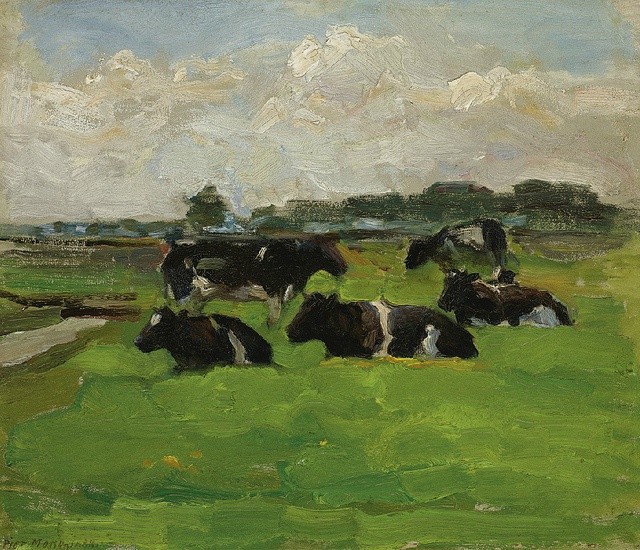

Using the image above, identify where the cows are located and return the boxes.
[161,232,348,329]
[403,218,509,272]
[438,269,573,328]
[285,290,479,359]
[134,306,273,371]
